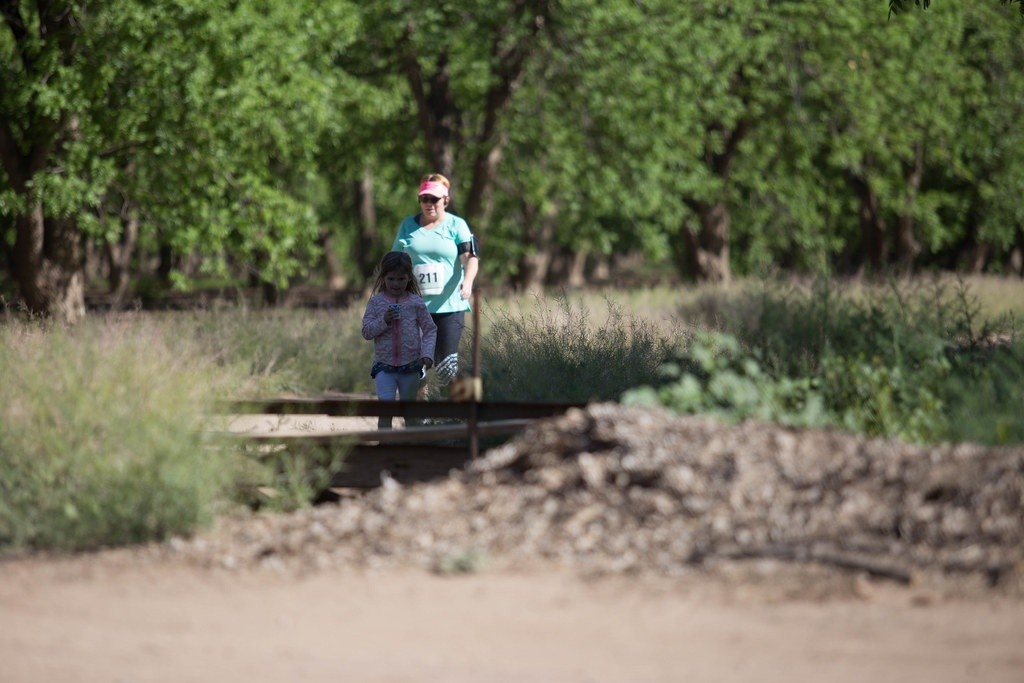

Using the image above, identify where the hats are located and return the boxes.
[417,181,448,198]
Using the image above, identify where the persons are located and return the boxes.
[391,174,481,424]
[362,252,437,428]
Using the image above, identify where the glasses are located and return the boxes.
[420,197,443,203]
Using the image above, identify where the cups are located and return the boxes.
[389,303,401,319]
